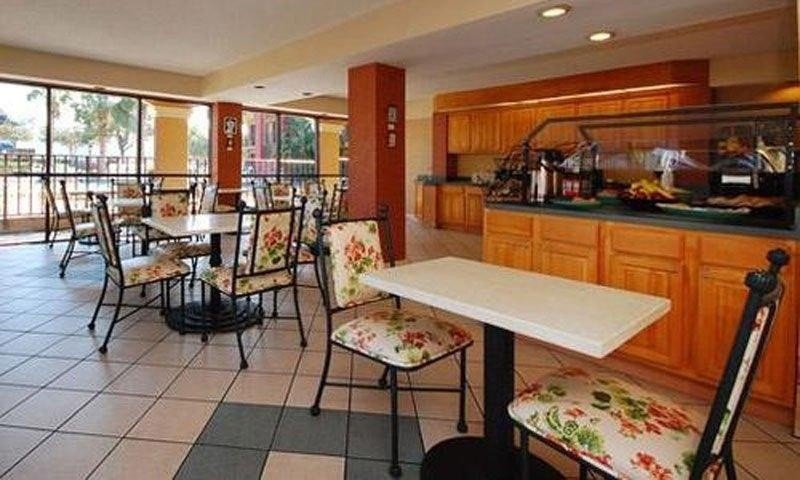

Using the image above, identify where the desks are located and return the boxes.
[86,199,161,260]
[358,256,672,480]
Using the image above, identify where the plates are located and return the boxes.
[656,203,752,221]
[554,193,622,211]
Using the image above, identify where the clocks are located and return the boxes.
[387,104,399,124]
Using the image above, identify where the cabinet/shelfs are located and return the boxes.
[447,94,669,158]
[484,207,601,290]
[608,225,800,396]
[438,192,483,233]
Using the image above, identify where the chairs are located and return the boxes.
[55,179,123,278]
[241,188,327,316]
[147,177,162,205]
[111,177,146,242]
[157,178,205,247]
[141,183,214,315]
[507,248,790,480]
[310,203,474,478]
[197,198,310,367]
[42,179,94,243]
[250,175,342,247]
[86,192,190,355]
